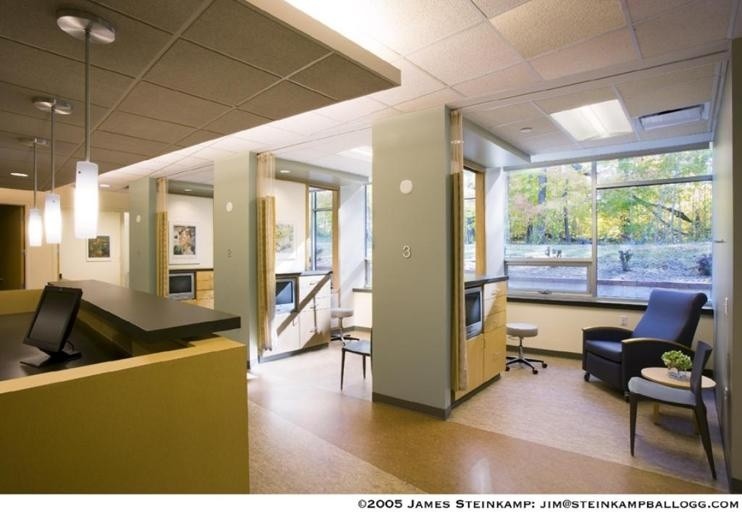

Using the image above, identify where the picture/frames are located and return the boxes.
[169,219,201,265]
[87,234,112,262]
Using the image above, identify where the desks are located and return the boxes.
[640,365,718,438]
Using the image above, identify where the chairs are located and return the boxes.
[582,289,708,404]
[340,326,372,389]
[628,340,714,480]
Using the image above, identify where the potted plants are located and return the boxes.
[662,349,694,377]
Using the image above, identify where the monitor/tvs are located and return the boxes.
[276,279,295,314]
[464,286,482,340]
[168,273,194,300]
[20,286,83,368]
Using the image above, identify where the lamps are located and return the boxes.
[20,137,49,247]
[54,11,116,239]
[33,97,72,243]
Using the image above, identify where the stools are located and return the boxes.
[505,323,548,374]
[331,308,360,344]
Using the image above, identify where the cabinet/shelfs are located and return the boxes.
[453,275,508,408]
[169,268,214,309]
[259,273,332,364]
